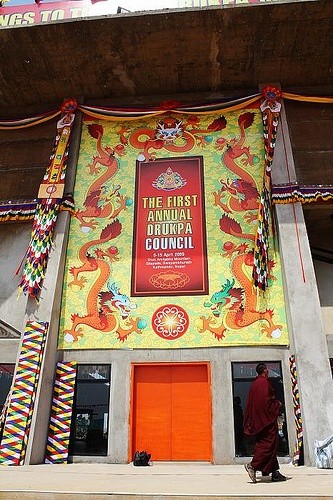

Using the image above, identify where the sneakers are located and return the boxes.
[271,471,286,482]
[244,463,257,483]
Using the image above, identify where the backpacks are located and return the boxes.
[133,450,154,466]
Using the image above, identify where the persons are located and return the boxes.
[233,396,247,452]
[242,362,289,484]
